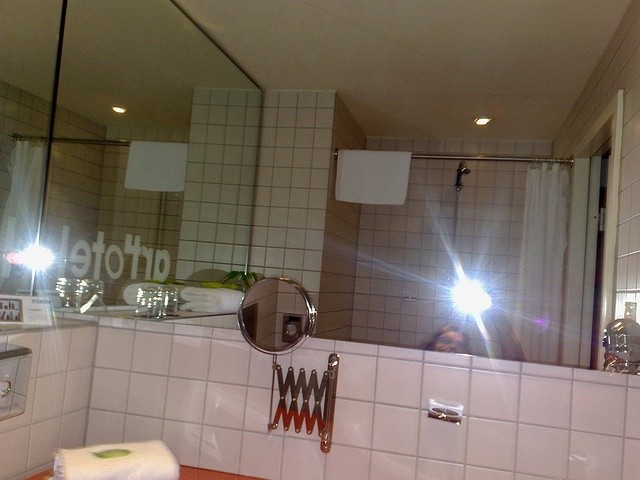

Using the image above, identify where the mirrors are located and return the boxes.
[238,277,316,354]
[3,2,265,333]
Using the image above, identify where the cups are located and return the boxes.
[135,284,164,317]
[80,280,104,307]
[164,286,181,316]
[55,277,79,309]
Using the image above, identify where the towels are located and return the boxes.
[123,141,190,195]
[335,147,412,207]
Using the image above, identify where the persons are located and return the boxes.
[418,285,525,364]
